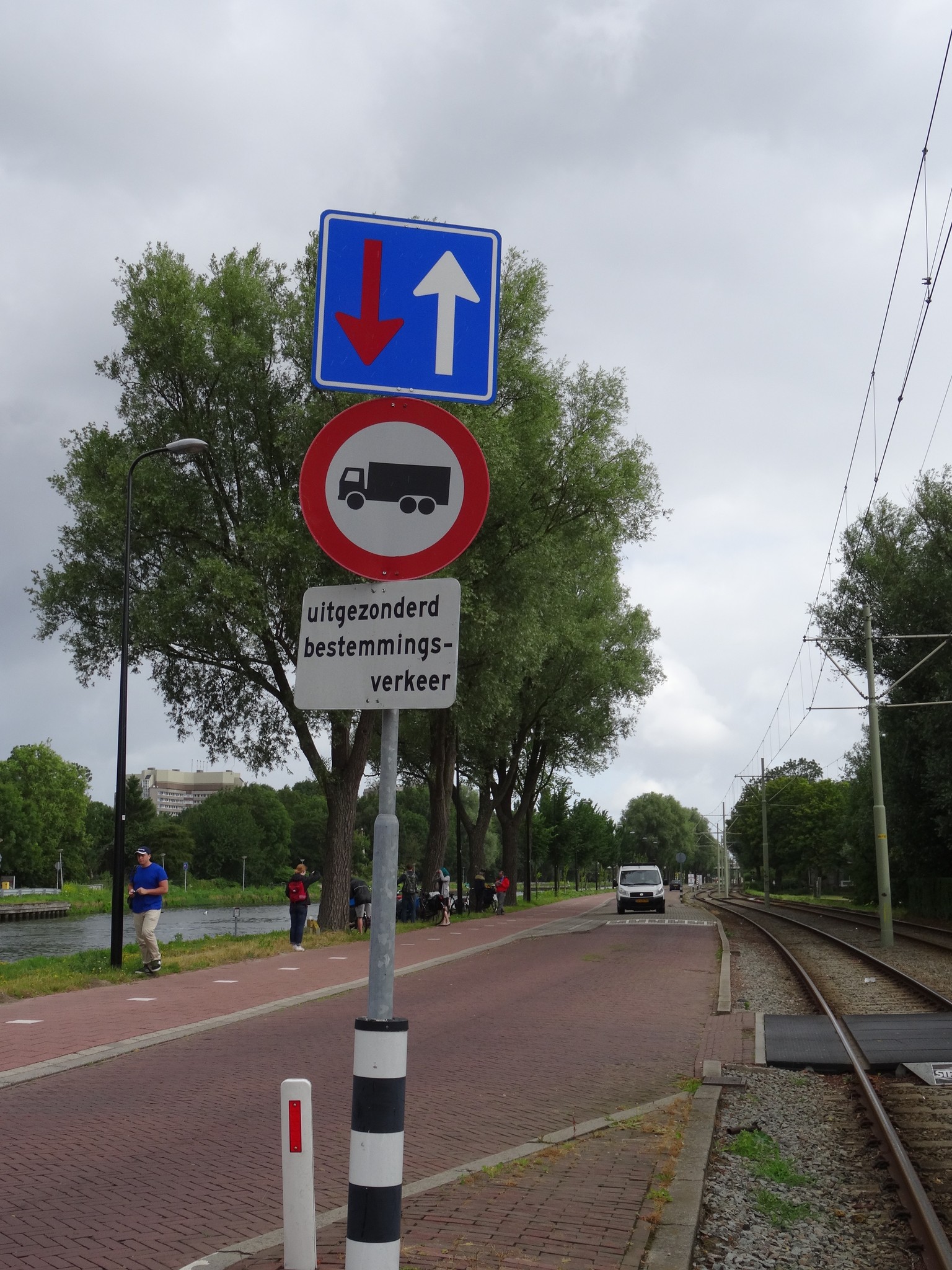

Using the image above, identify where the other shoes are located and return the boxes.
[501,912,504,915]
[497,913,501,915]
[293,946,297,949]
[439,923,446,926]
[295,945,305,951]
[446,922,451,926]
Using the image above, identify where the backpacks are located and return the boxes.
[289,879,307,902]
[404,872,417,894]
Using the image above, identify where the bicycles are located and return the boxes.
[349,886,371,934]
[396,885,445,925]
[463,885,496,914]
[448,891,470,919]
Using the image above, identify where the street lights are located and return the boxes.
[413,863,416,873]
[159,853,166,871]
[638,837,657,863]
[612,824,623,889]
[241,856,247,891]
[560,870,562,881]
[554,782,573,898]
[300,859,305,865]
[57,849,64,890]
[575,801,590,892]
[110,438,208,968]
[462,866,465,884]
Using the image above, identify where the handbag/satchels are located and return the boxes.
[127,865,139,909]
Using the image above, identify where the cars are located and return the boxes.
[669,880,681,892]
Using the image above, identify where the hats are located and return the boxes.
[134,846,151,855]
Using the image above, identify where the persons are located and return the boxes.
[495,871,510,915]
[127,846,168,976]
[285,864,320,952]
[432,868,451,927]
[474,869,487,913]
[396,864,419,923]
[350,877,372,935]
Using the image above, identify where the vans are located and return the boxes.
[613,865,665,914]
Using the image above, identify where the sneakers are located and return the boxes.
[135,964,152,974]
[151,959,161,972]
